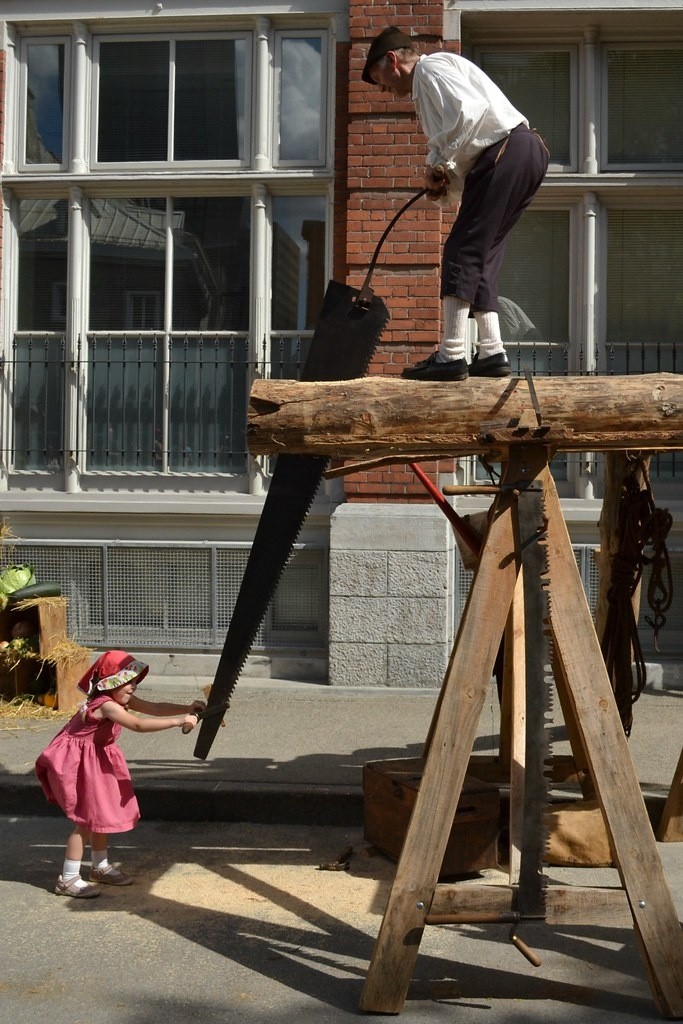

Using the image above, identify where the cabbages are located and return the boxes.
[0,562,36,595]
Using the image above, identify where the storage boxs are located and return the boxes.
[361,756,501,876]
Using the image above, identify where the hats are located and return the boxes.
[360,25,416,86]
[78,650,150,695]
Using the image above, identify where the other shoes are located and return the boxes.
[57,875,103,898]
[88,863,134,886]
[464,349,514,377]
[404,350,473,382]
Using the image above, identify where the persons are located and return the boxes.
[361,26,551,382]
[493,294,542,369]
[35,651,206,898]
